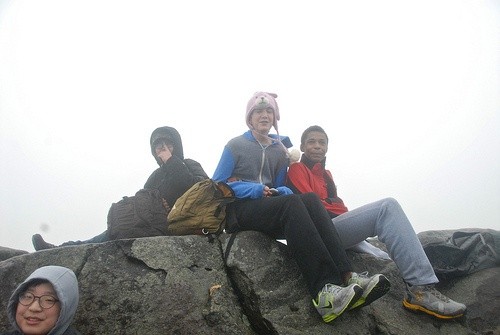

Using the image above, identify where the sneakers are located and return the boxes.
[312,282,363,323]
[348,272,392,311]
[402,284,467,318]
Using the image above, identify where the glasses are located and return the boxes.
[19,291,56,309]
[155,142,173,150]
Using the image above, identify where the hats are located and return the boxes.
[246,91,280,131]
[150,126,184,161]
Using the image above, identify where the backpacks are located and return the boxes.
[167,179,227,233]
[107,189,167,238]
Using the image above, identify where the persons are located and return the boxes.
[0,266,82,335]
[32,126,211,251]
[284,126,467,320]
[212,93,391,324]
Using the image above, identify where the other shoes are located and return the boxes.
[32,234,54,251]
